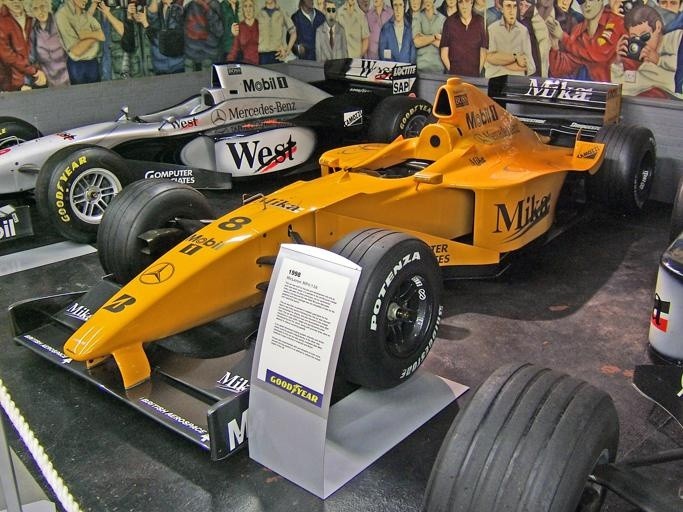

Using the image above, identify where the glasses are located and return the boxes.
[327,8,336,13]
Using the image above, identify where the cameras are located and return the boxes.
[620,0,644,15]
[621,36,646,62]
[132,0,147,13]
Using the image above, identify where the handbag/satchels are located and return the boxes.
[159,30,185,58]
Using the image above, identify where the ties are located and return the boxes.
[330,26,334,49]
